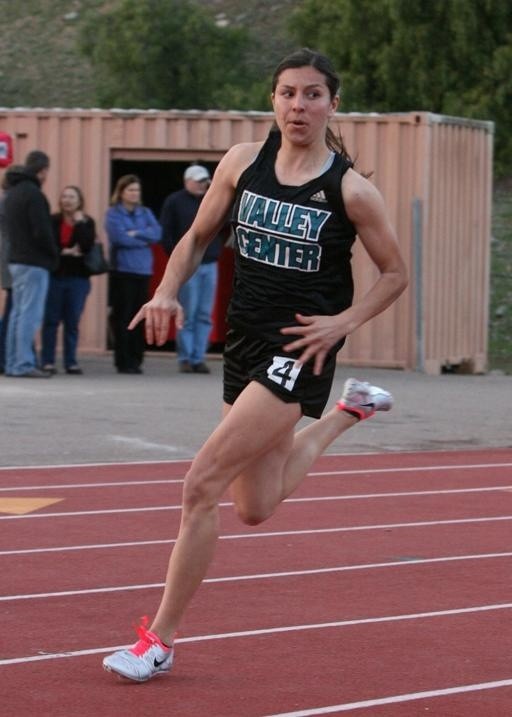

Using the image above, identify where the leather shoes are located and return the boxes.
[6,364,84,377]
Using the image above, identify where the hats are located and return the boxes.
[183,165,210,181]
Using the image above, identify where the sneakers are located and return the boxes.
[177,362,193,372]
[193,363,209,373]
[102,636,173,683]
[336,379,391,420]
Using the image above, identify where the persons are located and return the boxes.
[99,44,412,686]
[3,148,60,379]
[102,172,163,374]
[40,184,96,375]
[154,163,231,373]
[0,162,25,375]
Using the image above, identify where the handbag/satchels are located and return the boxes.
[73,243,106,280]
